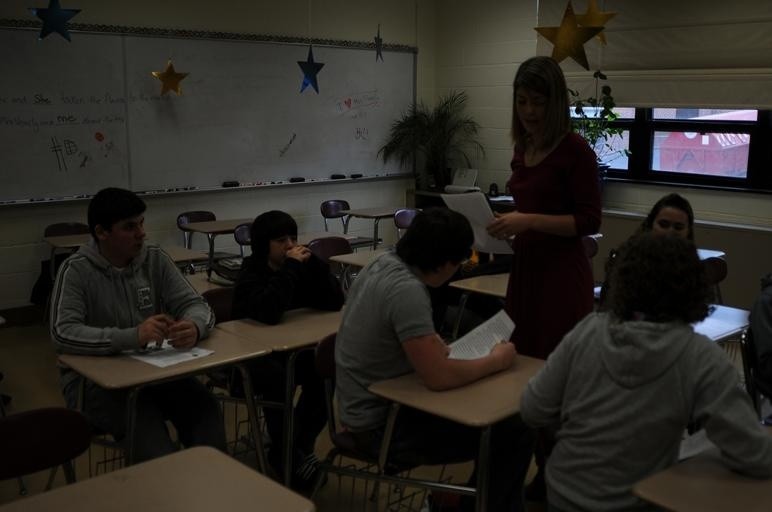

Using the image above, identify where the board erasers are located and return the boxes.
[330,174,345,179]
[350,174,362,177]
[290,177,305,182]
[222,181,240,187]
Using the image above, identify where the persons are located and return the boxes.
[230,210,344,492]
[336,209,517,468]
[522,228,772,511]
[632,193,697,240]
[46,187,226,464]
[485,56,602,501]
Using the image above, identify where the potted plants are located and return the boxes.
[566,71,633,191]
[376,87,486,192]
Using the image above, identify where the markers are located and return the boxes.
[374,173,392,177]
[242,181,284,186]
[142,186,200,195]
[310,179,320,181]
[1,193,94,204]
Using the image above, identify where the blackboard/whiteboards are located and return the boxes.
[126,23,419,195]
[2,24,126,208]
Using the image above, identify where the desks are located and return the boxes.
[414,190,517,213]
[634,446,771,510]
[2,446,317,511]
[365,355,549,511]
[691,246,752,364]
[448,254,510,342]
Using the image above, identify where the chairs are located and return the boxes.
[39,199,420,487]
[1,407,93,486]
[310,332,422,510]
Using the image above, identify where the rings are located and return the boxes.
[502,232,509,239]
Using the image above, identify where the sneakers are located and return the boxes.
[267,444,327,487]
